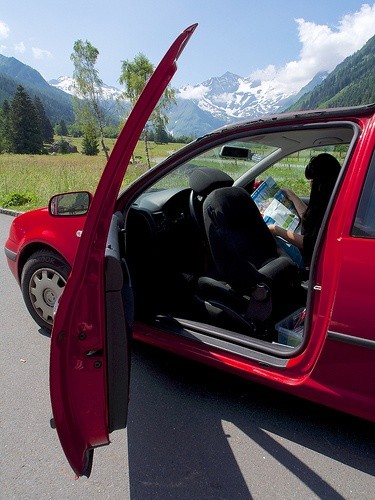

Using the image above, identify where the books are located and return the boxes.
[250,175,303,248]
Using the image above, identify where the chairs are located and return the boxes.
[186,167,302,332]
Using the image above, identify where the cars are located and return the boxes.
[3,23,375,481]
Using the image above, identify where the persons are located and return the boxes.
[267,153,342,272]
